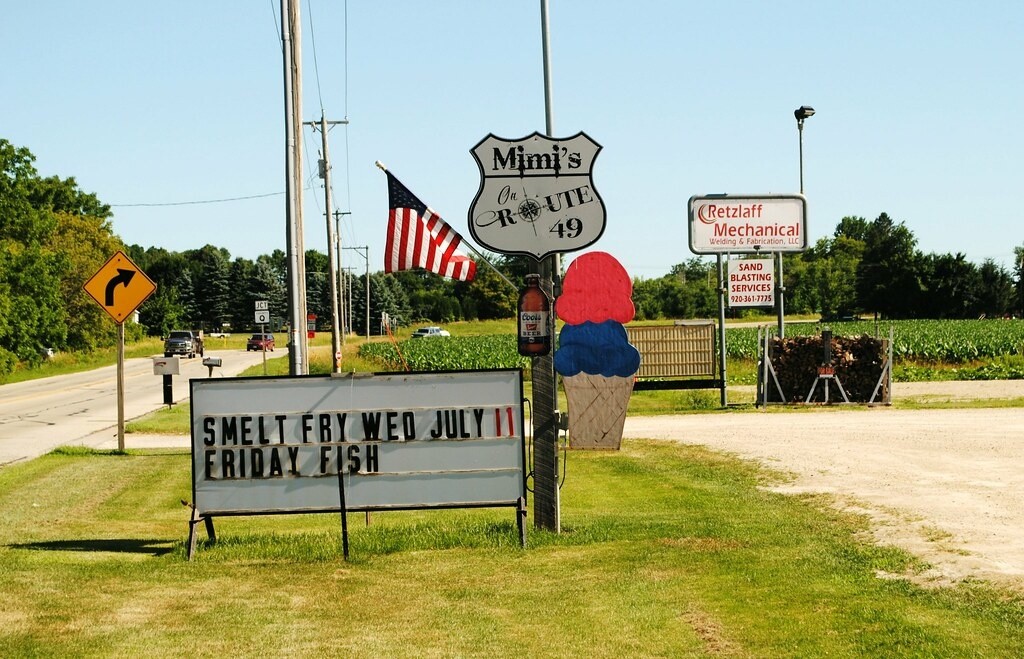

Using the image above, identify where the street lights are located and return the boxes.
[793,104,815,195]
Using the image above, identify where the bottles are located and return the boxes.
[517,273,551,356]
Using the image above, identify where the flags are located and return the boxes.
[384,173,476,281]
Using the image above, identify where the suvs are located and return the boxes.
[411,327,442,338]
[247,334,275,352]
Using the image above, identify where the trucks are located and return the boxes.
[161,331,205,357]
